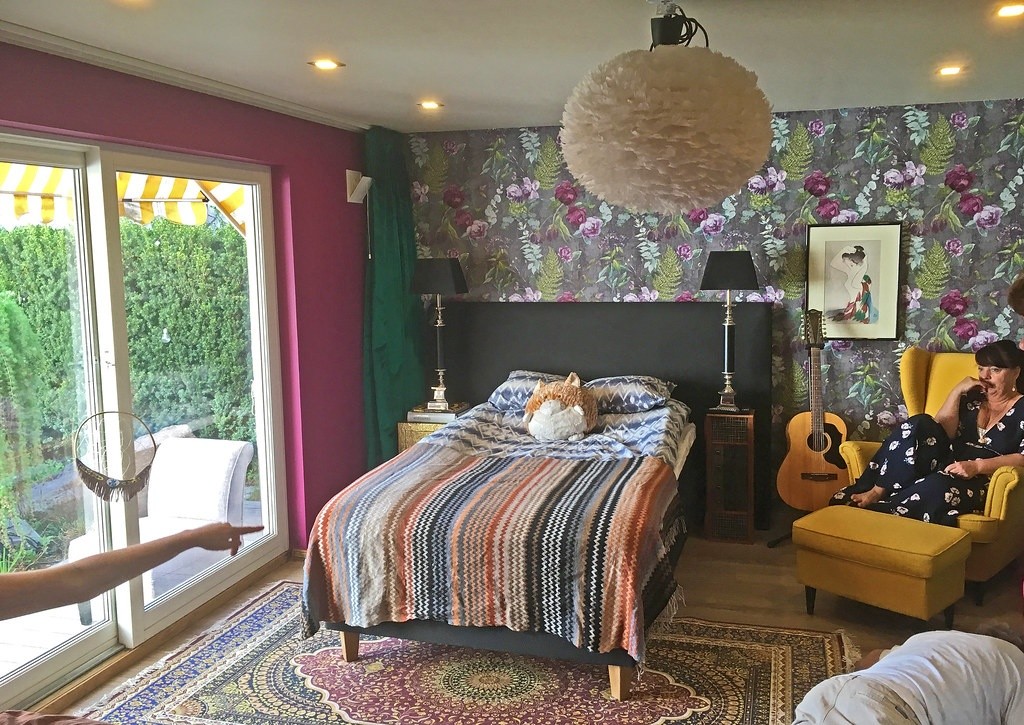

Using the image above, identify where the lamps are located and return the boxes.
[558,0,774,216]
[700,251,759,412]
[410,257,469,408]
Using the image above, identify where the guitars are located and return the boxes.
[772,308,851,514]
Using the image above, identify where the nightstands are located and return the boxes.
[397,421,446,455]
[705,410,757,544]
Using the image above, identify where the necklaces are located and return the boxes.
[978,399,1009,443]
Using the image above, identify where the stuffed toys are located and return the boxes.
[522,372,599,441]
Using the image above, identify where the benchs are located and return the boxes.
[793,505,971,632]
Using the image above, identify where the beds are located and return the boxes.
[298,298,771,702]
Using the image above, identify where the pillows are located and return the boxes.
[582,375,677,414]
[482,370,589,412]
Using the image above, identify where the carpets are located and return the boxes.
[76,580,859,725]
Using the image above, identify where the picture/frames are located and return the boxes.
[805,222,901,341]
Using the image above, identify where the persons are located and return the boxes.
[791,623,1024,725]
[0,521,265,622]
[850,340,1024,522]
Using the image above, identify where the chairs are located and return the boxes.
[839,347,1024,607]
[67,436,254,607]
